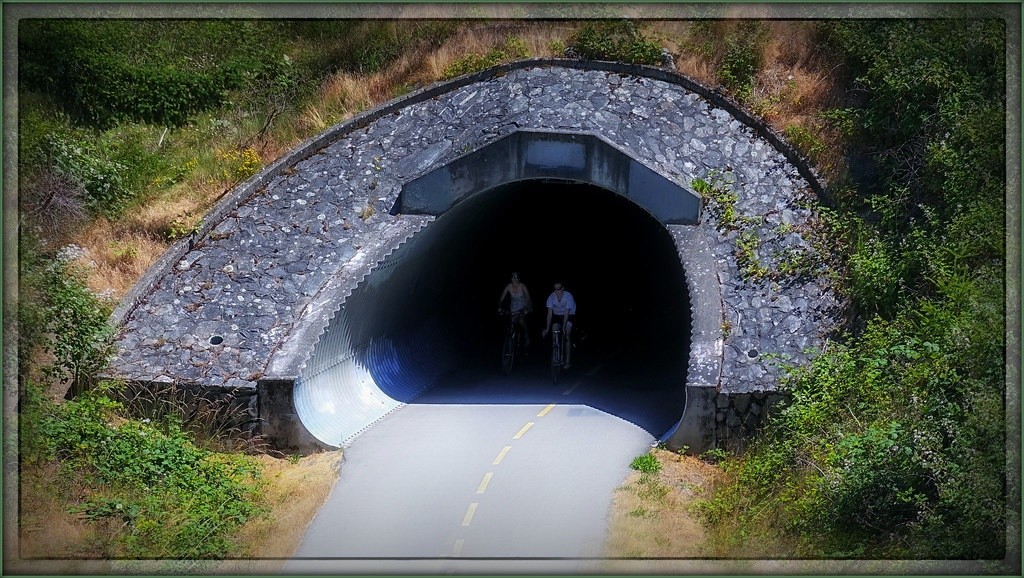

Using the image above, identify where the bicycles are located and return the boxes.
[549,330,572,385]
[500,310,528,375]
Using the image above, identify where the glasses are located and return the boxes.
[553,286,562,291]
[511,275,518,279]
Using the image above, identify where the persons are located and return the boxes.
[542,282,576,369]
[497,272,533,347]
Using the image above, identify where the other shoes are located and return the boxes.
[563,362,571,369]
